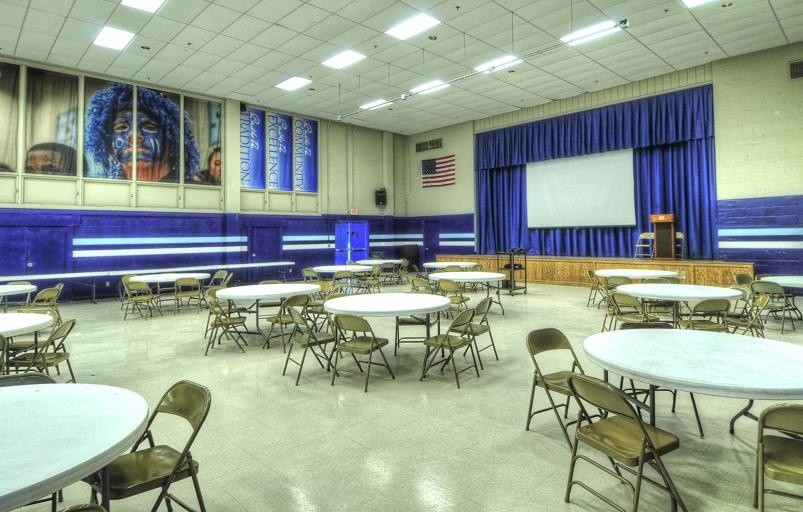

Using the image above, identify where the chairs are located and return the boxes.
[633,232,655,261]
[674,231,686,260]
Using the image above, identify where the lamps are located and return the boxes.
[336,116,344,121]
[401,93,412,100]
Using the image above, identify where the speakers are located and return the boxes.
[375,191,386,205]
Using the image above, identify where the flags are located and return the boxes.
[421,155,455,189]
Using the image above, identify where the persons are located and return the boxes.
[199,147,221,186]
[84,81,203,185]
[26,142,88,177]
